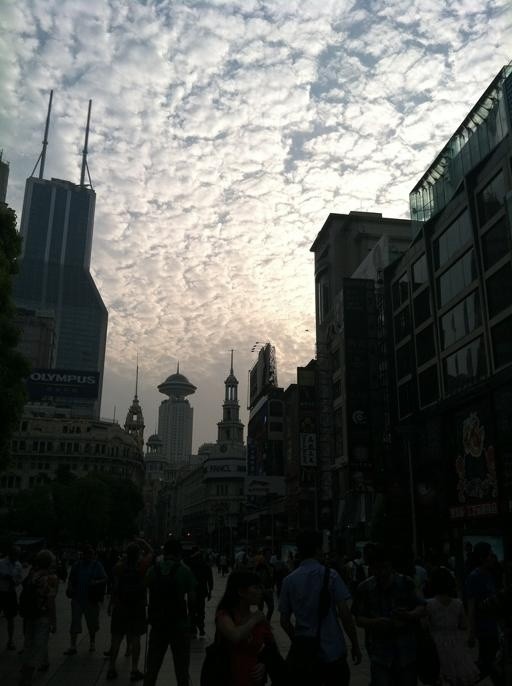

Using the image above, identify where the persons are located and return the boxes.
[1,519,512,685]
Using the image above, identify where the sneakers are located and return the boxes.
[131,670,144,682]
[106,670,118,678]
[102,650,110,656]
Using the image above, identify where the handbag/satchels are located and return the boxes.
[285,636,329,685]
